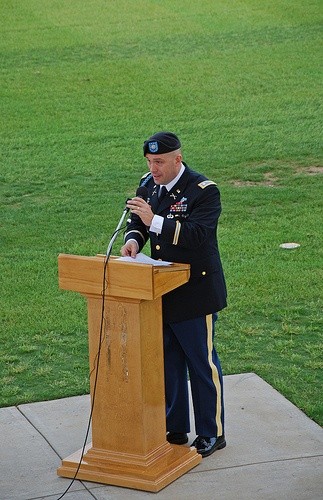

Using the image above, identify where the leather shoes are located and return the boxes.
[165,431,190,445]
[190,434,226,458]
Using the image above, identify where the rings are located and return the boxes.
[137,207,140,210]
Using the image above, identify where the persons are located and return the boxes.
[120,131,228,458]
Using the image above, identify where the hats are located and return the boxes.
[142,132,182,157]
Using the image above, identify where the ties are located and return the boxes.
[158,186,170,207]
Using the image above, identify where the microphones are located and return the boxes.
[131,185,148,224]
